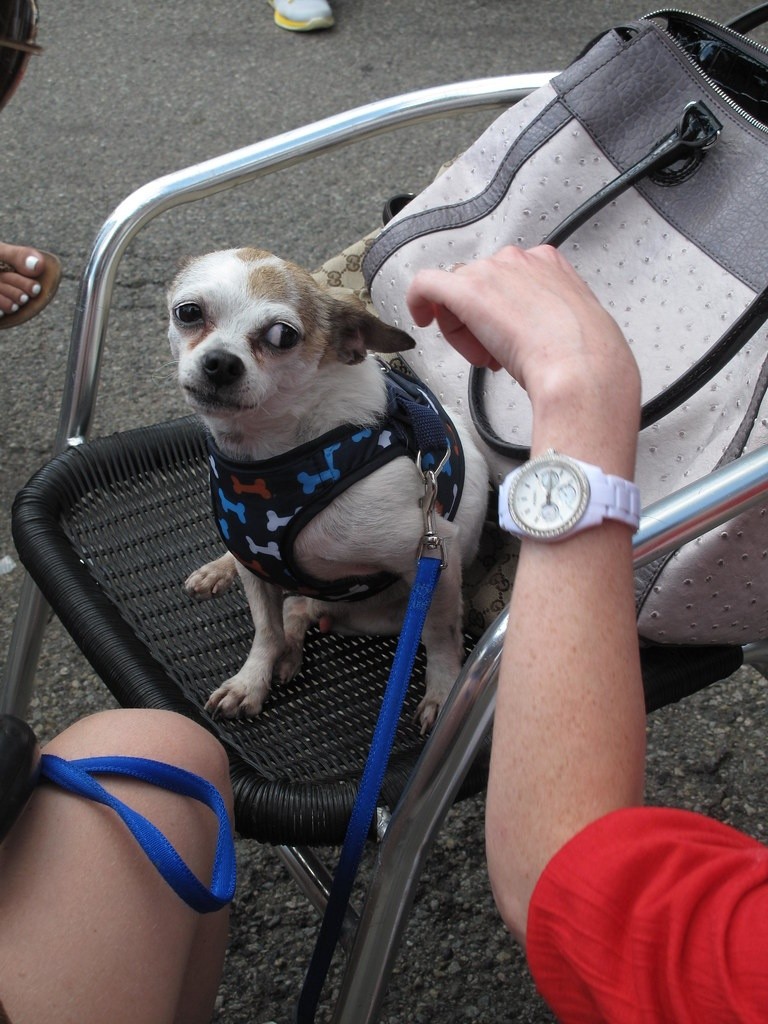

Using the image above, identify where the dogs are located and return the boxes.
[162,242,494,737]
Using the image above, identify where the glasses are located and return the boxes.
[0,0,42,114]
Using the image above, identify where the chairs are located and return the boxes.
[0,72,768,1024]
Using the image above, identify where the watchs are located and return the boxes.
[499,450,642,542]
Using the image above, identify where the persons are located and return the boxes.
[0,707,237,1024]
[408,244,768,1024]
[268,0,337,31]
[0,243,61,329]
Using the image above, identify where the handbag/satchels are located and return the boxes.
[312,3,768,647]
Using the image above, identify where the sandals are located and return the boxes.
[0,241,62,330]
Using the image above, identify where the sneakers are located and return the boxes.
[268,0,335,31]
